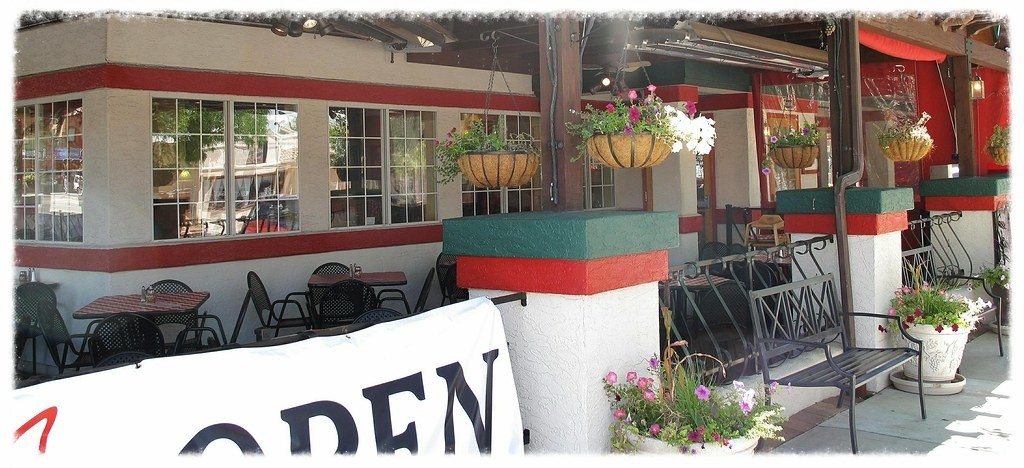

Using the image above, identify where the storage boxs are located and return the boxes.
[929,163,960,180]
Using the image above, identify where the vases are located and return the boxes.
[989,148,1011,166]
[879,139,931,161]
[769,146,818,169]
[624,430,761,456]
[459,151,537,190]
[585,135,670,170]
[902,324,972,383]
[992,283,1012,327]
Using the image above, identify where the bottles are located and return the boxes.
[28,268,36,282]
[350,264,356,278]
[141,286,145,302]
[146,285,155,303]
[19,271,27,282]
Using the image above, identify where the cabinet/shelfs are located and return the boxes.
[744,215,790,248]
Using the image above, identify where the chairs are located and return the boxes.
[14,253,467,392]
[659,241,795,351]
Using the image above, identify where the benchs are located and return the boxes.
[236,197,300,234]
[902,245,1005,374]
[747,273,927,455]
[152,198,191,240]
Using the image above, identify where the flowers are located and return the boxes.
[878,280,992,333]
[435,112,540,184]
[760,120,825,176]
[874,111,935,150]
[982,123,1010,153]
[602,353,786,456]
[564,87,716,175]
[967,257,1010,292]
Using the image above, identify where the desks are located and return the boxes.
[306,272,407,329]
[660,273,731,334]
[38,212,82,240]
[73,291,209,372]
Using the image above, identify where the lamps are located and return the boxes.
[969,72,986,101]
[269,19,335,39]
[590,74,628,94]
